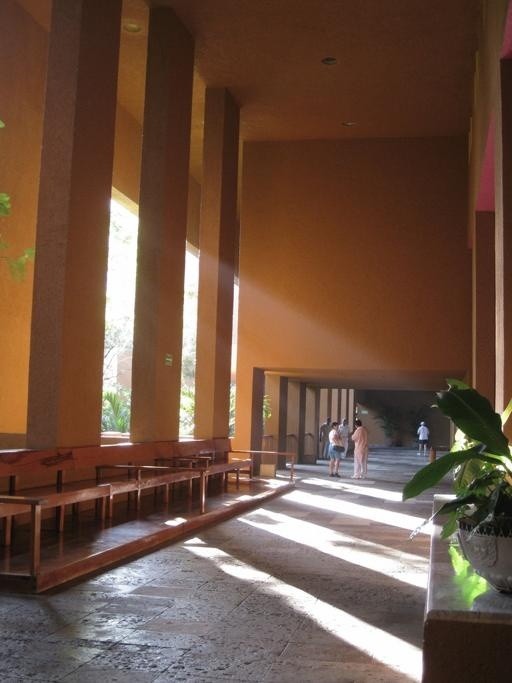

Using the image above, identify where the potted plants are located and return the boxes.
[374,404,403,448]
[408,391,430,449]
[403,378,512,597]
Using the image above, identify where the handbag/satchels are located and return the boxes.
[332,445,345,452]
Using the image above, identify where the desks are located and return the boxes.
[422,494,512,683]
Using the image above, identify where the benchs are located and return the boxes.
[0,438,253,577]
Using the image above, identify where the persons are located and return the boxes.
[337,419,353,459]
[416,421,429,455]
[328,421,343,477]
[320,417,332,459]
[349,419,368,478]
